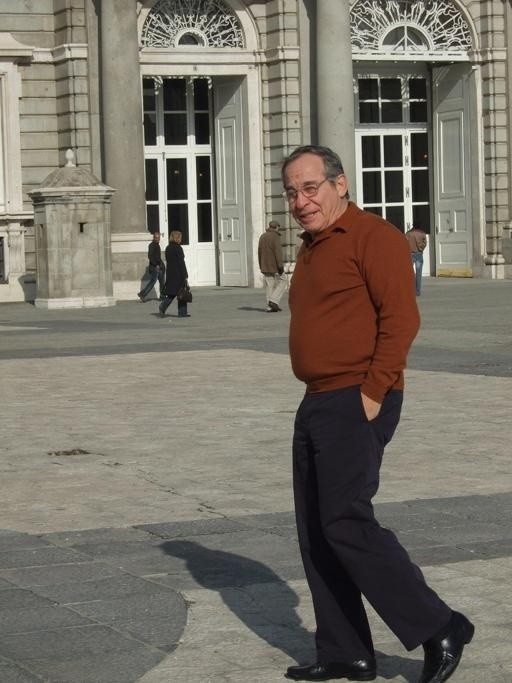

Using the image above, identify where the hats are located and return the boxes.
[269,220,281,226]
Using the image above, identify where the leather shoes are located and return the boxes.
[286,660,376,680]
[418,610,475,683]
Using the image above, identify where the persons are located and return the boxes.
[406,221,426,296]
[281,145,474,683]
[159,230,190,317]
[138,232,166,303]
[259,221,287,312]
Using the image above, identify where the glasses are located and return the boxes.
[281,178,329,204]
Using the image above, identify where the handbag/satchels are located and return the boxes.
[178,280,193,303]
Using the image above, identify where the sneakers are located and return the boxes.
[157,305,191,318]
[266,302,281,311]
[137,292,147,303]
